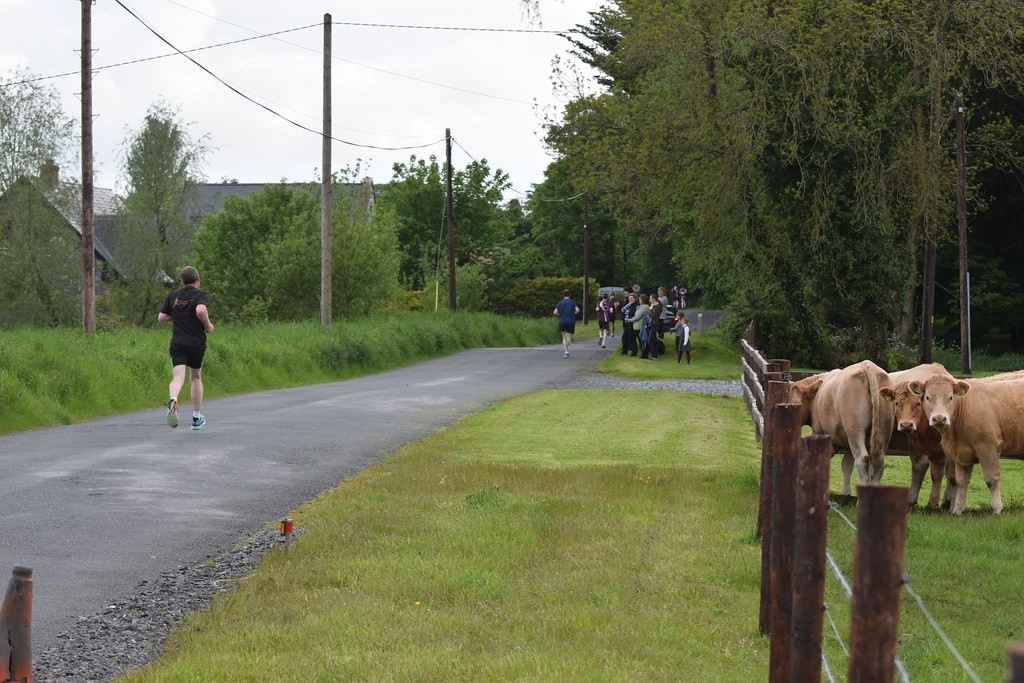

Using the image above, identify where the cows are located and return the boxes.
[878,370,1024,517]
[786,360,954,513]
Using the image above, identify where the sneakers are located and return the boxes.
[165,398,179,429]
[190,415,206,430]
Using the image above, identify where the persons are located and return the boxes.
[554,289,580,357]
[606,294,619,337]
[678,316,691,364]
[596,294,613,348]
[658,287,668,338]
[646,294,666,358]
[622,288,652,358]
[158,266,214,430]
[670,312,684,351]
[680,286,687,308]
[671,285,680,308]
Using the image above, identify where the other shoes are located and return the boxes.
[565,333,571,346]
[650,357,658,361]
[598,337,603,346]
[563,353,570,358]
[602,344,606,349]
[640,355,649,359]
[611,334,614,339]
[620,352,628,355]
[630,354,637,356]
